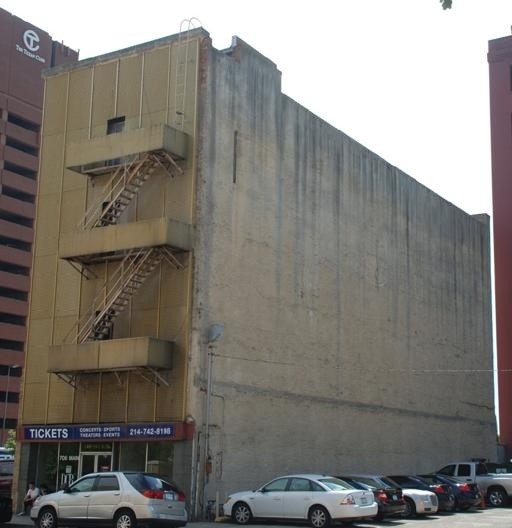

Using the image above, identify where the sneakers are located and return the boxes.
[18,512,26,516]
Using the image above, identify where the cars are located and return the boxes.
[220,471,484,527]
[30,470,190,528]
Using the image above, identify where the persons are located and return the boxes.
[17,482,41,518]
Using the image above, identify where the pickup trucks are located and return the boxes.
[437,462,512,506]
